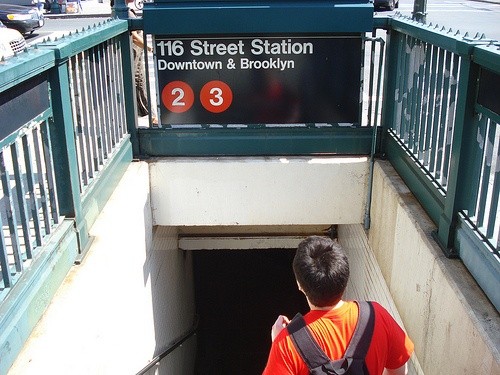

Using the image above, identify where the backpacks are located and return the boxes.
[286,300,375,375]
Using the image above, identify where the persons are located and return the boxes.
[262,235,415,375]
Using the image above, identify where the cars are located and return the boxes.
[0,23,27,62]
[0,3,44,39]
